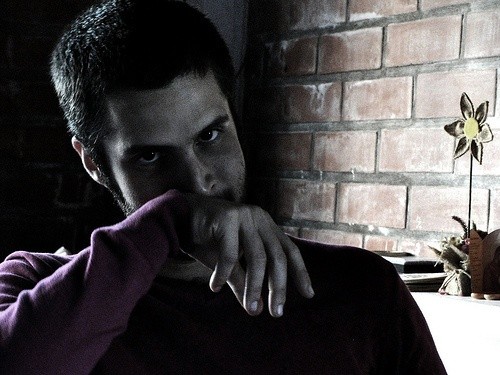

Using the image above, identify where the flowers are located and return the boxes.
[432,93,494,295]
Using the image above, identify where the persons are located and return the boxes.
[1,0,446,374]
